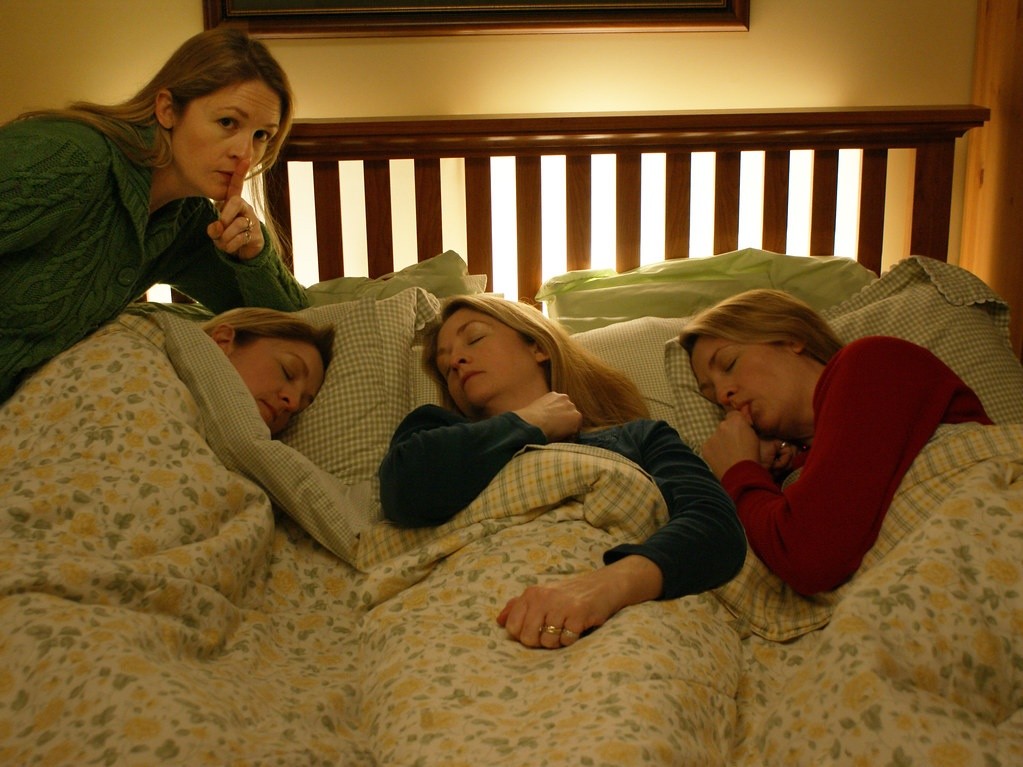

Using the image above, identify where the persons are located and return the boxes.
[0,27,311,409]
[380,293,747,650]
[679,289,997,602]
[198,308,336,436]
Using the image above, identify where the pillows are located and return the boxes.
[266,247,1022,497]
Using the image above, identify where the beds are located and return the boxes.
[0,102,1023,767]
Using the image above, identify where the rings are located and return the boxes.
[244,231,251,244]
[245,217,254,231]
[562,629,579,639]
[781,442,786,448]
[541,625,563,634]
